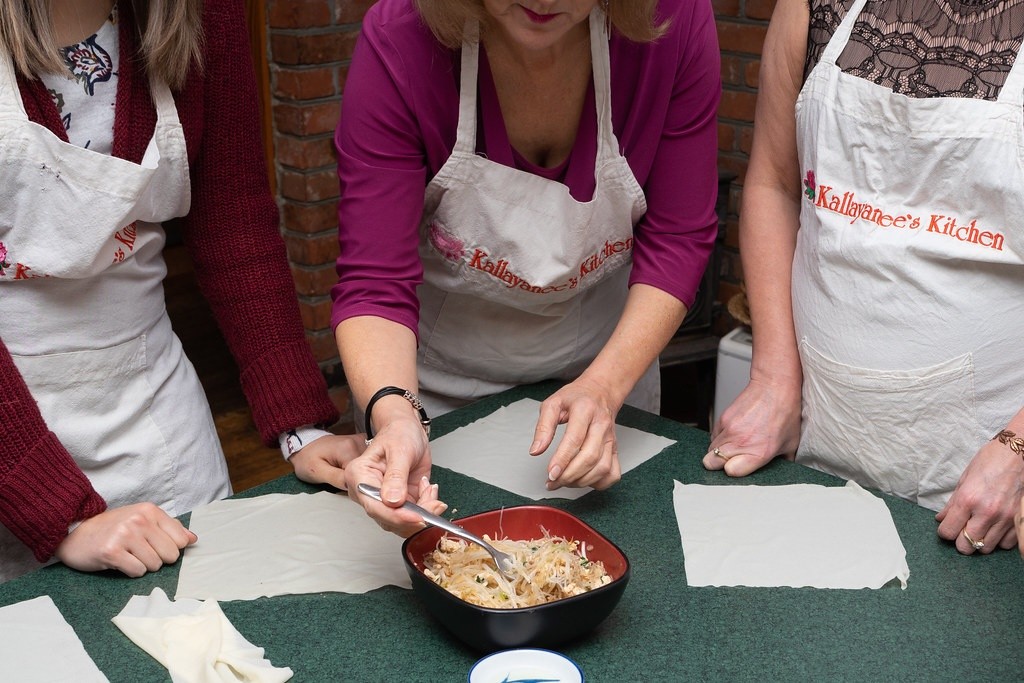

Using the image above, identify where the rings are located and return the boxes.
[714,448,730,460]
[963,527,985,550]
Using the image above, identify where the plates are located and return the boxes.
[467,648,582,682]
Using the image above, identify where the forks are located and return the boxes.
[357,483,522,580]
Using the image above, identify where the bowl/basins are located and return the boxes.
[401,504,632,656]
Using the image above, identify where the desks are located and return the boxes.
[1,380,1024,683]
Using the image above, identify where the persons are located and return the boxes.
[701,1,1024,556]
[1,0,370,577]
[332,1,722,539]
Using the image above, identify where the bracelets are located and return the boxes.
[365,387,431,448]
[990,428,1024,461]
